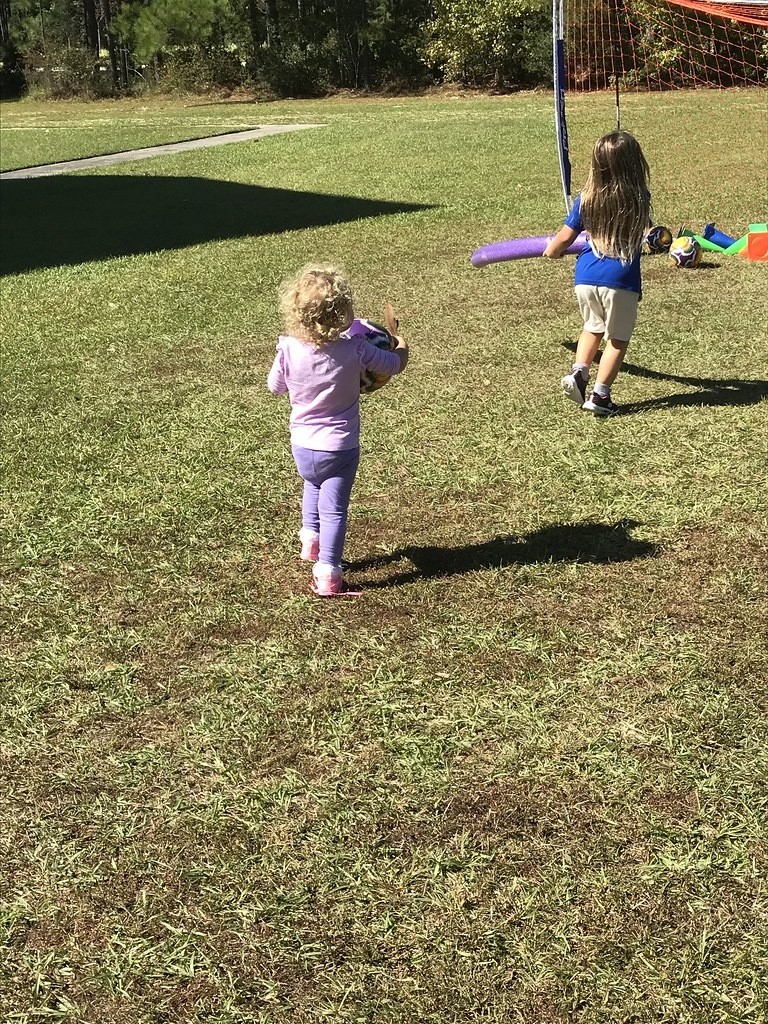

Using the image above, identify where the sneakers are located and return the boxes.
[582,390,619,415]
[563,368,589,405]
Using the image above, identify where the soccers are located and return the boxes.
[669,236,702,269]
[641,226,672,255]
[339,318,396,395]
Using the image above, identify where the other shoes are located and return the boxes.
[313,564,343,596]
[299,529,320,561]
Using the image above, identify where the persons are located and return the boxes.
[543,132,652,417]
[266,262,409,595]
